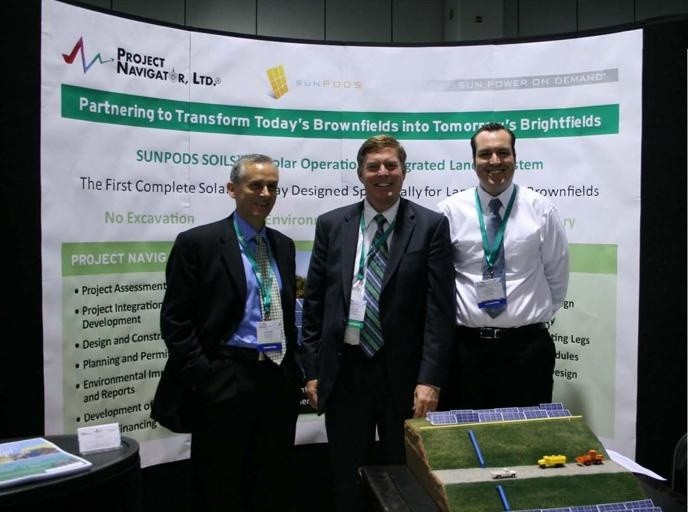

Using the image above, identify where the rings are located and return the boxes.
[423,407,429,411]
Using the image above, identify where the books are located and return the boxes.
[0,436,93,488]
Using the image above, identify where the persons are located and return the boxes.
[430,120,572,410]
[145,150,304,511]
[294,133,461,510]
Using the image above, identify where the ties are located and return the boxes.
[252,236,287,368]
[482,199,506,319]
[359,216,389,359]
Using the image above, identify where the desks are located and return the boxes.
[0,434,140,512]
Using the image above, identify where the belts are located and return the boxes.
[342,343,387,358]
[205,346,268,362]
[457,323,549,340]
